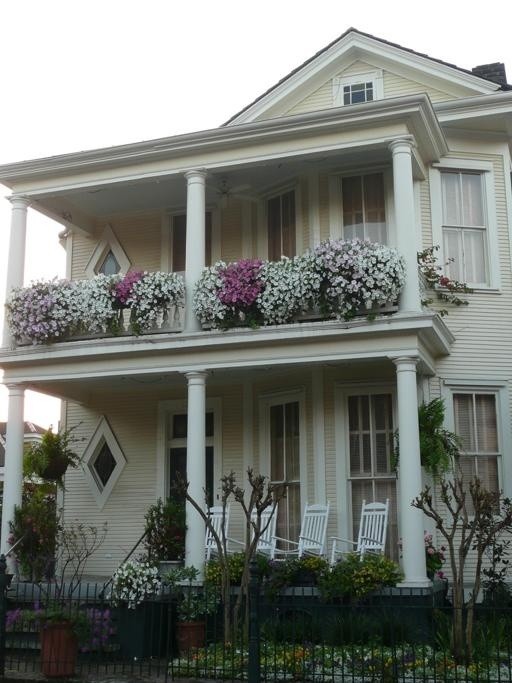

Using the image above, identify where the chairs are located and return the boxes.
[205,497,390,571]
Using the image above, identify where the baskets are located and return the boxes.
[39,458,68,481]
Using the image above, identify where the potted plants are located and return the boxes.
[164,564,221,657]
[23,419,89,492]
[387,394,467,480]
[144,493,188,572]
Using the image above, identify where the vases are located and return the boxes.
[116,598,151,662]
[39,621,77,678]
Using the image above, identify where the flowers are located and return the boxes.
[6,588,115,652]
[104,559,163,610]
[5,243,475,347]
